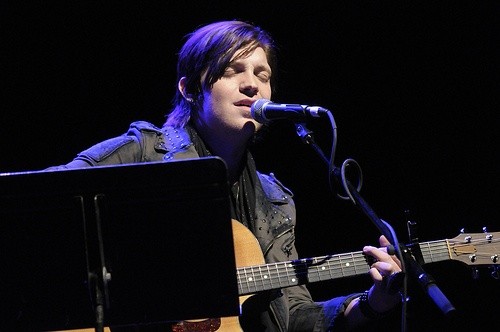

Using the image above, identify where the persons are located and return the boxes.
[42,21,403,332]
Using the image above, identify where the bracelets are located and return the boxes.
[358,290,383,319]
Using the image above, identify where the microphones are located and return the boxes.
[250,99,329,124]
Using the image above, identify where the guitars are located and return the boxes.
[51,218,500,332]
